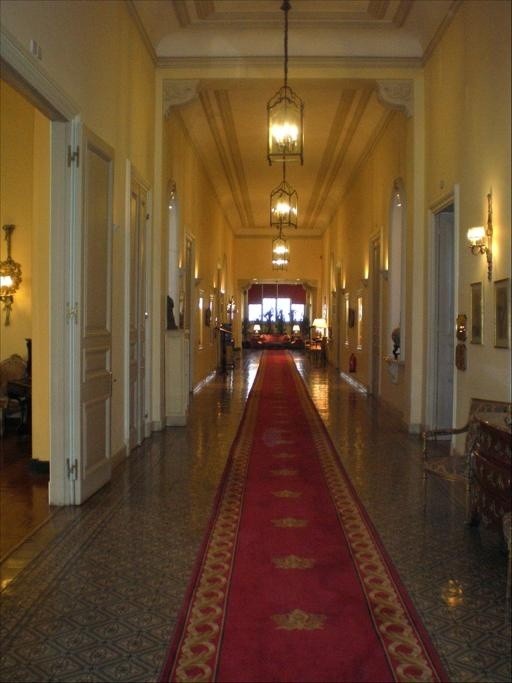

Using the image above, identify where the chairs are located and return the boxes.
[0,354,28,437]
[422,398,512,599]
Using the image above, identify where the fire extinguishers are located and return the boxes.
[349,353,356,372]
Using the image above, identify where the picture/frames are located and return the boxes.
[493,278,512,349]
[470,280,483,344]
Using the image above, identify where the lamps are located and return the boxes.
[272,227,287,254]
[467,192,492,279]
[270,162,298,229]
[0,224,22,325]
[266,6,304,167]
